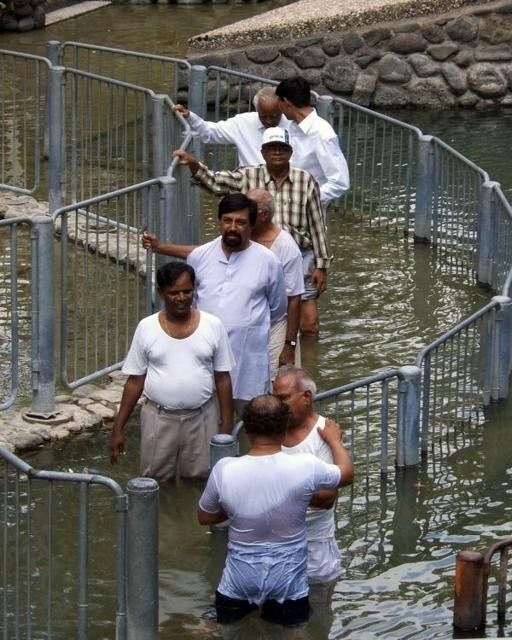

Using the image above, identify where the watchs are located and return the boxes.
[285,339,297,347]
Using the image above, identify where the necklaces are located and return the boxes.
[163,311,193,338]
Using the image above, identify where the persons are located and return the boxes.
[140,189,306,394]
[171,86,291,168]
[274,76,351,226]
[172,125,333,336]
[272,367,345,585]
[107,260,236,481]
[185,193,289,428]
[195,394,355,629]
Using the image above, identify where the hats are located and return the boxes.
[260,125,293,151]
